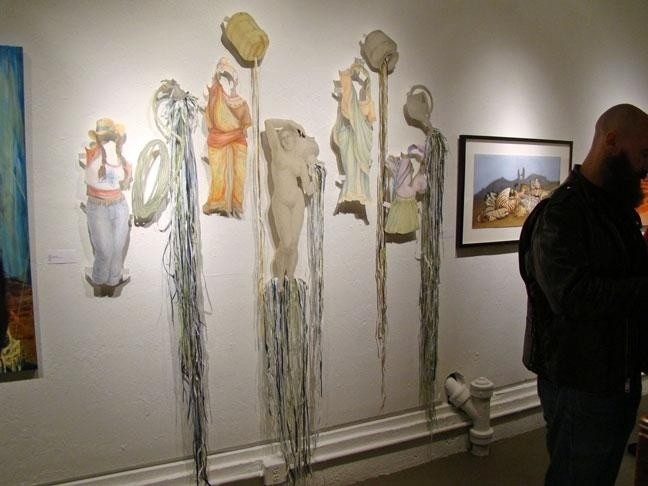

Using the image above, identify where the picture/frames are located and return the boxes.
[453,134,575,249]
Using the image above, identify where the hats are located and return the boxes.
[87,118,126,140]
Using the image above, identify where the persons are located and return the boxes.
[264,117,321,292]
[382,144,431,235]
[77,117,134,288]
[517,102,648,485]
[201,55,252,219]
[329,56,377,205]
